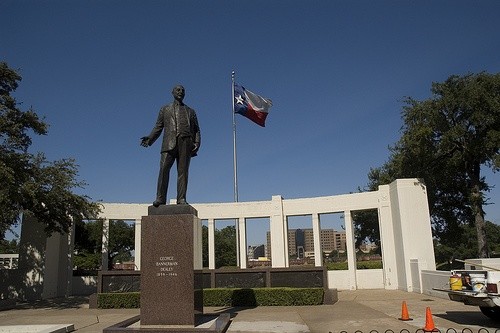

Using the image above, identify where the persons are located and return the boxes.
[140,85,201,207]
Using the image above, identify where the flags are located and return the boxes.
[233,80,272,127]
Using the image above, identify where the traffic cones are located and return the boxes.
[399,301,412,322]
[419,306,438,332]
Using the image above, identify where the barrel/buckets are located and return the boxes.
[449,274,463,291]
[469,273,486,293]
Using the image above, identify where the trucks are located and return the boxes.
[433,255,500,321]
